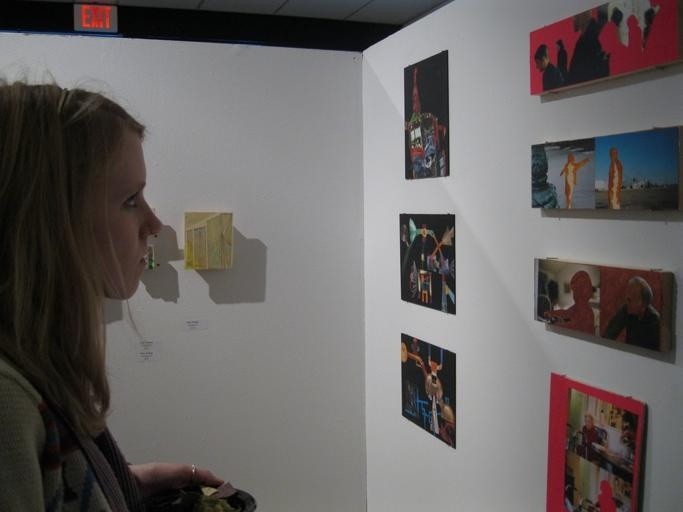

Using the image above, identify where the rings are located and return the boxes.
[190,463,199,482]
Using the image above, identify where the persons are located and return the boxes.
[558,150,591,208]
[600,274,662,350]
[543,268,596,337]
[2,73,255,512]
[567,17,613,87]
[607,149,623,211]
[574,405,601,452]
[554,40,567,83]
[532,45,565,93]
